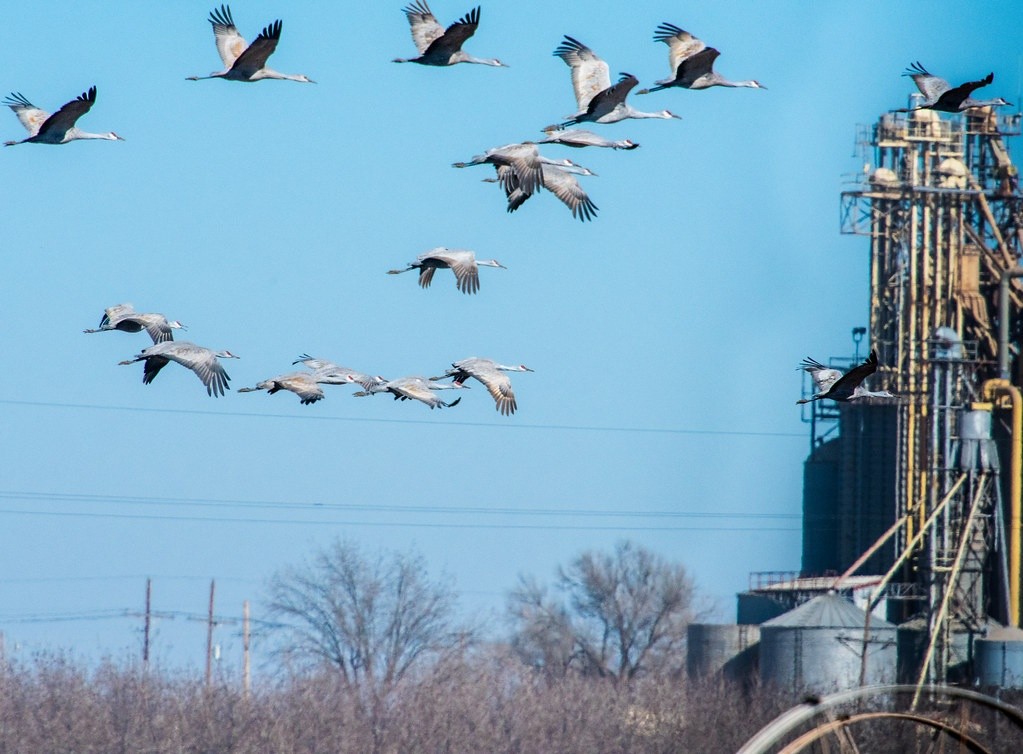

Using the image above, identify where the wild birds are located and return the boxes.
[0,84,128,150]
[382,0,508,69]
[384,246,507,297]
[540,35,683,130]
[636,21,767,94]
[83,303,192,347]
[521,123,642,152]
[791,349,905,405]
[451,142,600,224]
[113,339,241,401]
[236,354,534,417]
[183,4,315,88]
[887,60,1014,127]
